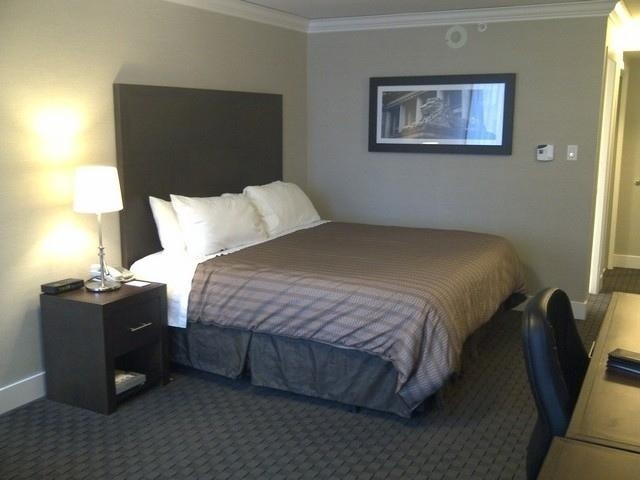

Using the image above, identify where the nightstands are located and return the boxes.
[39,278,167,416]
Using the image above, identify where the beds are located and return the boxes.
[130,219,527,412]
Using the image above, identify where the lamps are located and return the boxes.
[71,162,126,292]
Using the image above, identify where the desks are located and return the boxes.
[537,291,640,480]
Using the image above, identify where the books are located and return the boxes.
[607,348,639,374]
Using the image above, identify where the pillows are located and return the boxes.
[147,179,324,263]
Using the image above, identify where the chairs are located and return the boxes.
[520,285,591,479]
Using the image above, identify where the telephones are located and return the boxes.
[91,265,135,283]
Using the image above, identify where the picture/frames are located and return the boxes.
[367,72,516,155]
